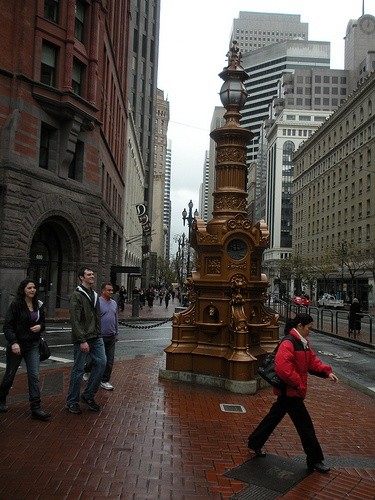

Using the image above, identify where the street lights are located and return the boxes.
[178,231,187,301]
[181,199,200,307]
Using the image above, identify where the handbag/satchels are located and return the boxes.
[258,337,286,388]
[39,337,51,361]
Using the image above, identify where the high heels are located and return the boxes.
[306,456,330,471]
[248,442,266,456]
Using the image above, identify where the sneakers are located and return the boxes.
[0,406,11,413]
[64,403,81,414]
[83,363,88,381]
[100,382,114,390]
[31,410,52,420]
[81,394,100,410]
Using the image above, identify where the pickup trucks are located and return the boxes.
[318,295,345,308]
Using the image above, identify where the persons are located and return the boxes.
[119,286,127,312]
[350,298,362,334]
[247,312,338,472]
[132,286,175,309]
[0,279,52,420]
[82,282,119,391]
[65,267,106,414]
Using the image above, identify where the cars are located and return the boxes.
[293,296,309,307]
